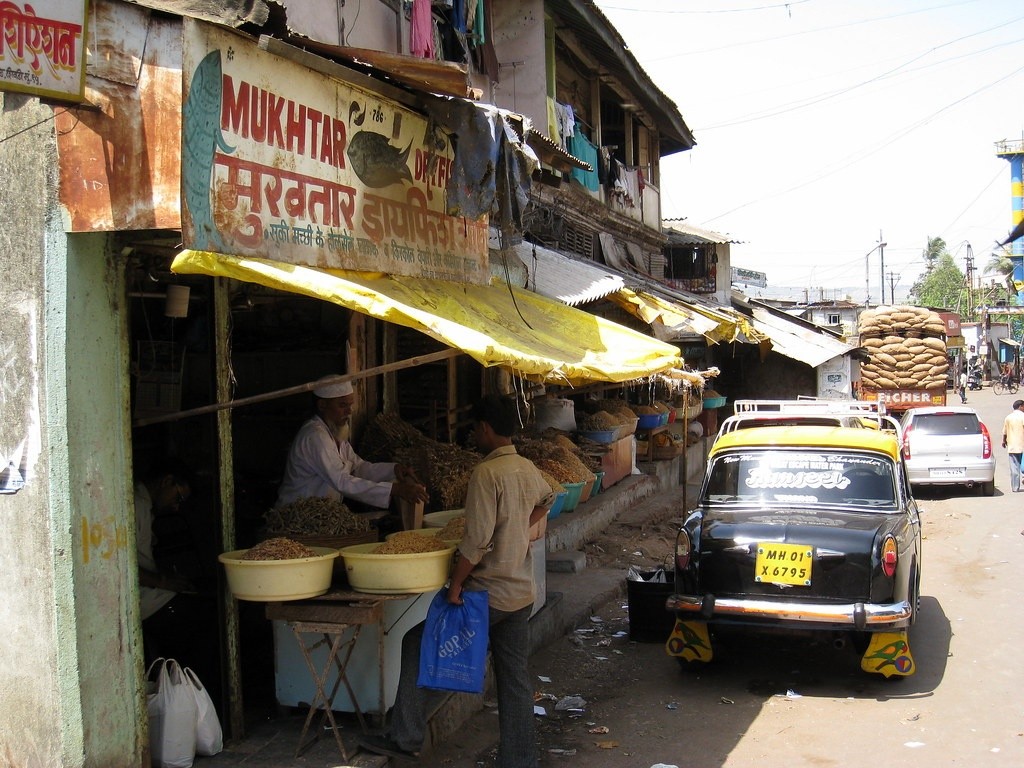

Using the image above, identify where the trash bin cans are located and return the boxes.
[626,570,677,644]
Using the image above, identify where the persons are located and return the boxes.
[356,396,557,768]
[959,368,967,404]
[1002,400,1024,492]
[134,456,222,687]
[1000,362,1013,388]
[262,374,430,539]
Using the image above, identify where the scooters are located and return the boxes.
[967,369,983,391]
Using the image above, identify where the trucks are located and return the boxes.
[858,380,946,428]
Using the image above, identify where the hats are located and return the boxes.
[313,375,353,398]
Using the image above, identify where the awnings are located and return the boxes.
[998,338,1021,346]
[171,241,681,385]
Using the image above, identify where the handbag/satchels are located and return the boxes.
[143,657,197,768]
[183,667,223,757]
[415,590,490,693]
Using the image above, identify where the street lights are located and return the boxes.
[865,243,887,309]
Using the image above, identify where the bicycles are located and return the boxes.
[993,373,1019,395]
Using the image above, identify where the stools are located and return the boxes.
[287,623,371,761]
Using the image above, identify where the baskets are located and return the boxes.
[256,524,378,576]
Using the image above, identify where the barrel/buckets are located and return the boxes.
[624,570,677,643]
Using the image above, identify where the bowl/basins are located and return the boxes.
[386,527,463,545]
[545,471,606,520]
[423,508,467,527]
[578,396,727,446]
[218,546,339,601]
[339,541,457,595]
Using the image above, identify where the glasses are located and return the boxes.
[173,482,184,503]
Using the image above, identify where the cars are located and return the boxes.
[664,427,922,681]
[903,406,994,497]
[714,398,903,440]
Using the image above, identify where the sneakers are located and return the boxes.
[359,732,417,762]
